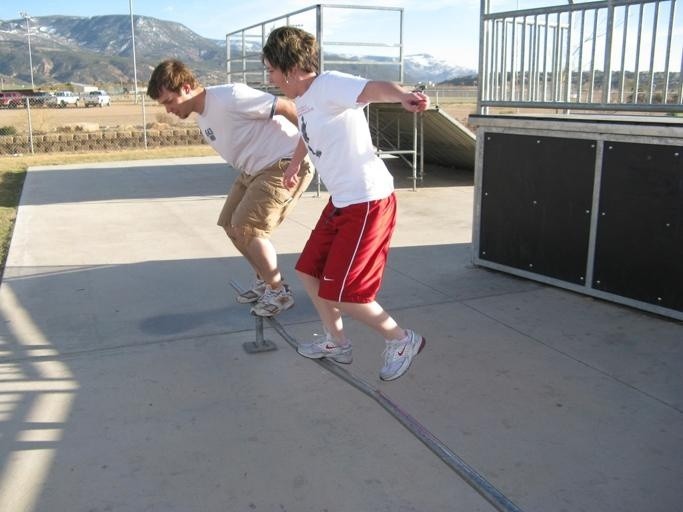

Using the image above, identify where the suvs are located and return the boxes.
[0,91,21,109]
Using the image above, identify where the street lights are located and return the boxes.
[16,7,37,91]
[416,80,436,97]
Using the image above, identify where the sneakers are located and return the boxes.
[250,283,294,317]
[236,272,291,304]
[379,329,425,381]
[297,324,353,364]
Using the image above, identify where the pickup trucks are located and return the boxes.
[21,92,58,109]
[43,90,80,109]
[82,89,111,108]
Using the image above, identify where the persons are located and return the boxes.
[262,26,429,381]
[147,60,315,318]
